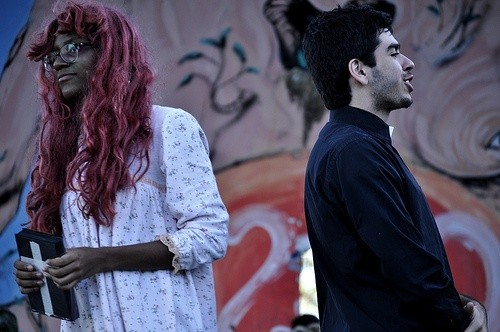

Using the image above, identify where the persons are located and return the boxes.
[301,3,490,332]
[13,1,231,332]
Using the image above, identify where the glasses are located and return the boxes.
[42,41,93,72]
